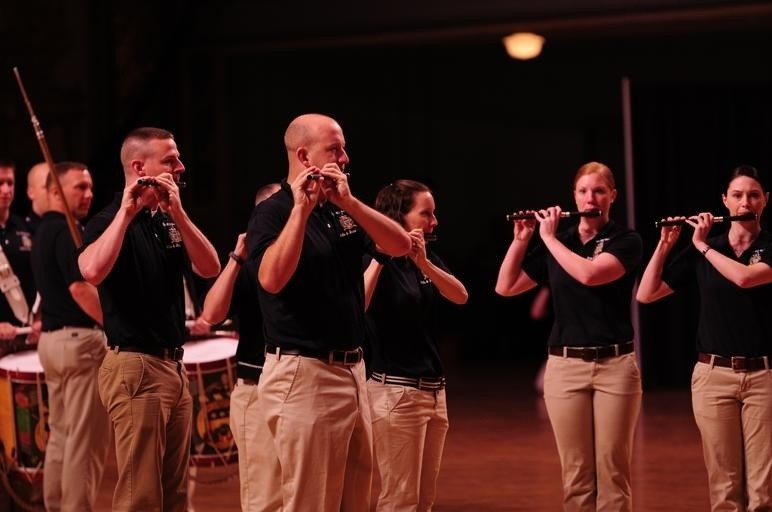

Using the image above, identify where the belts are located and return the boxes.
[111,345,184,360]
[267,345,360,365]
[370,371,447,390]
[550,342,633,362]
[699,353,772,371]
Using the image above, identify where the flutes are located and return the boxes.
[138,178,185,188]
[308,173,349,180]
[424,232,436,241]
[656,214,757,228]
[507,211,602,220]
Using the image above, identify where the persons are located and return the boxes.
[495,162,643,512]
[200,181,280,512]
[77,128,221,512]
[32,162,113,512]
[0,162,42,356]
[24,160,53,235]
[636,165,772,512]
[247,113,411,512]
[364,179,469,512]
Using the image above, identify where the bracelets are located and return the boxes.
[702,245,712,256]
[229,252,244,266]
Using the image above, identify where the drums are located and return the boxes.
[182,336,238,468]
[1,350,46,511]
